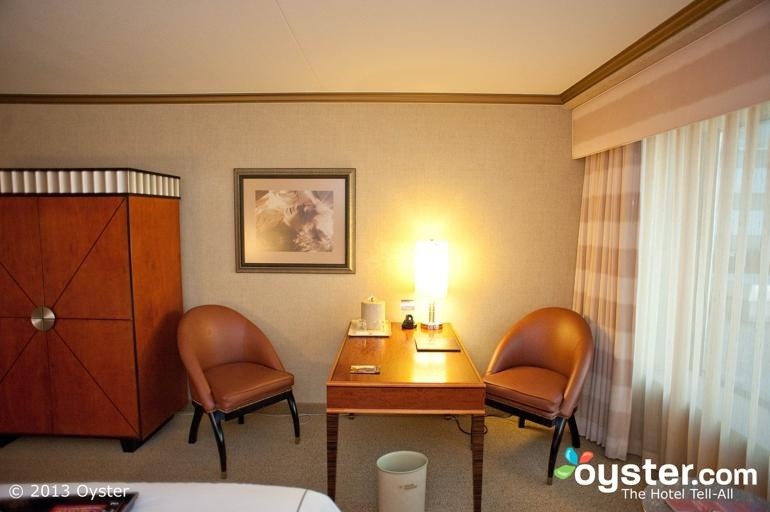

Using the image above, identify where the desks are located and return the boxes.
[1,483,339,512]
[642,476,770,512]
[326,321,487,512]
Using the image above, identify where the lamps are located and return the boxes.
[412,242,456,328]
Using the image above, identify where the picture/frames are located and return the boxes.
[234,166,357,276]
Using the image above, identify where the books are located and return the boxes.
[0,490,139,512]
[416,336,462,352]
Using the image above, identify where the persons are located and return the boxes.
[255,189,333,254]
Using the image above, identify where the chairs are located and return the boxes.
[177,304,299,480]
[482,307,594,486]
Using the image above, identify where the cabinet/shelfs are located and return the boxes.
[0,167,181,452]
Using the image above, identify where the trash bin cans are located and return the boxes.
[376,451,428,512]
